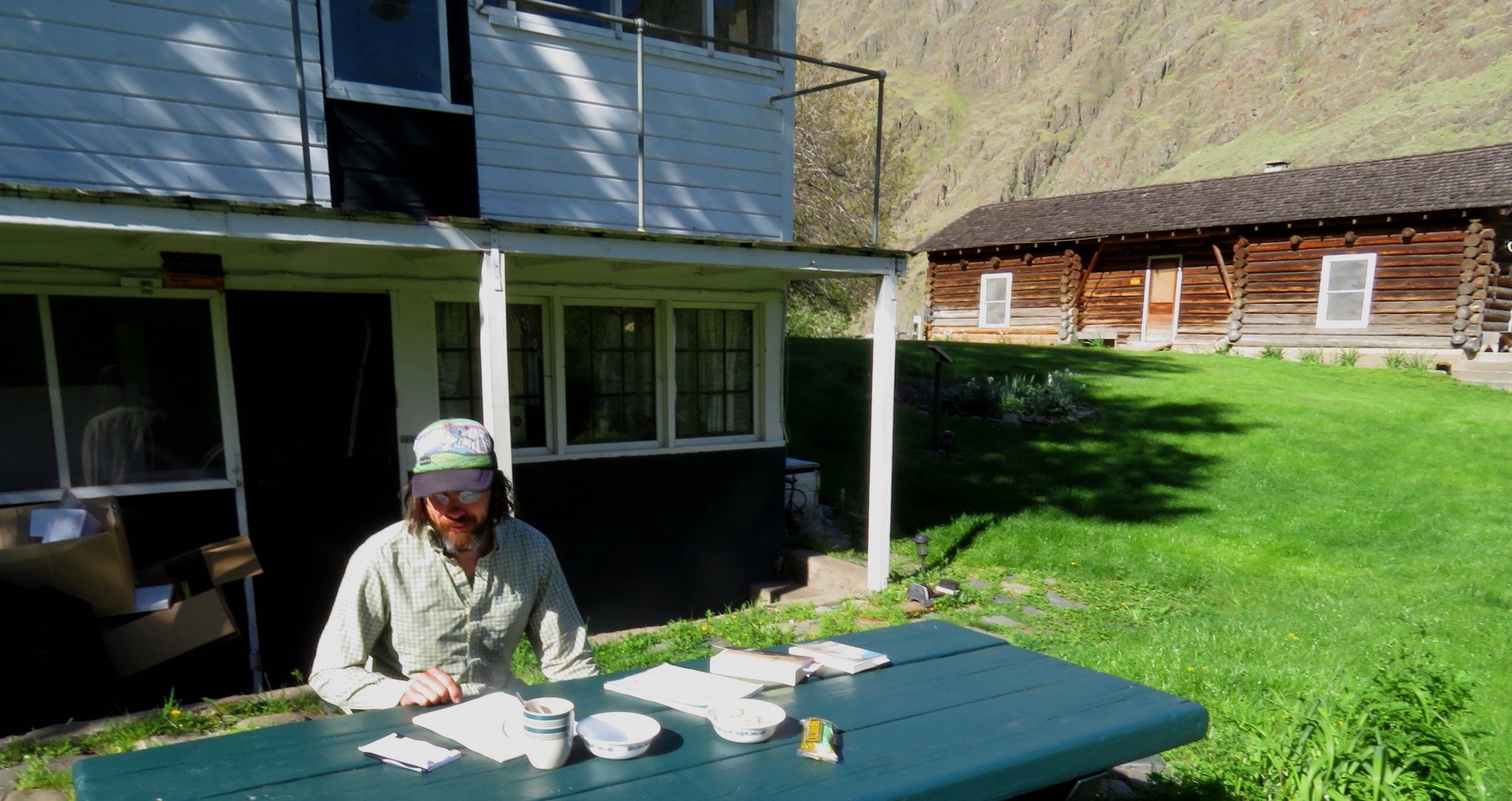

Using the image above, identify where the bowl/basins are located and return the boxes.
[704,698,786,742]
[575,712,661,760]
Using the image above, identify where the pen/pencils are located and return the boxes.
[364,752,428,774]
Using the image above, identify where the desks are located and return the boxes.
[72,619,1207,801]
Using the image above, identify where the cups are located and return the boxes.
[522,696,574,771]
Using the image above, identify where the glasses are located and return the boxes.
[423,490,482,510]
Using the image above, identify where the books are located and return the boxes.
[709,642,820,689]
[357,731,464,773]
[788,639,891,676]
[605,661,767,721]
[410,690,580,764]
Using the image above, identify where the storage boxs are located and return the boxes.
[0,496,263,678]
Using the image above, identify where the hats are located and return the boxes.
[412,418,497,499]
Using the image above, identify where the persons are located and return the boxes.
[306,418,601,707]
